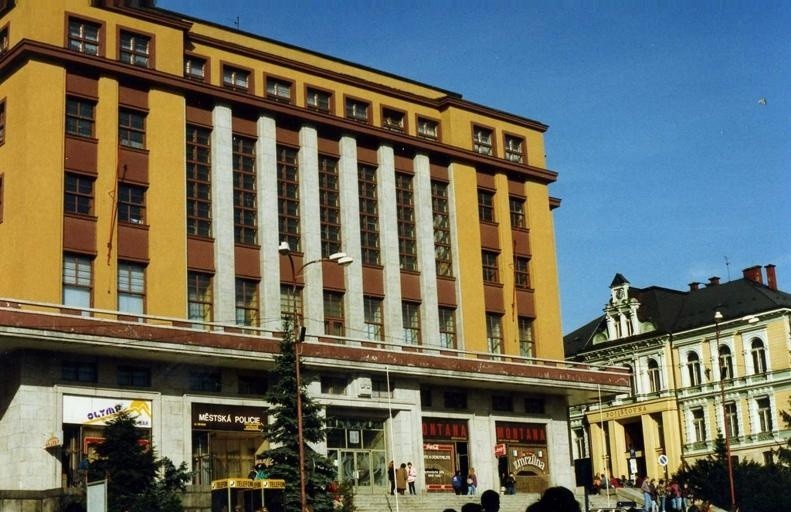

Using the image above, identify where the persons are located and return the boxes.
[461,503,484,512]
[406,462,418,495]
[395,463,408,495]
[387,460,396,495]
[467,467,478,494]
[481,490,501,511]
[526,486,582,512]
[591,472,714,512]
[452,470,465,496]
[506,472,517,495]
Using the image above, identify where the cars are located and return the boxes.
[589,501,647,512]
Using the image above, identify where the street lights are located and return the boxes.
[279,242,354,512]
[714,311,760,504]
